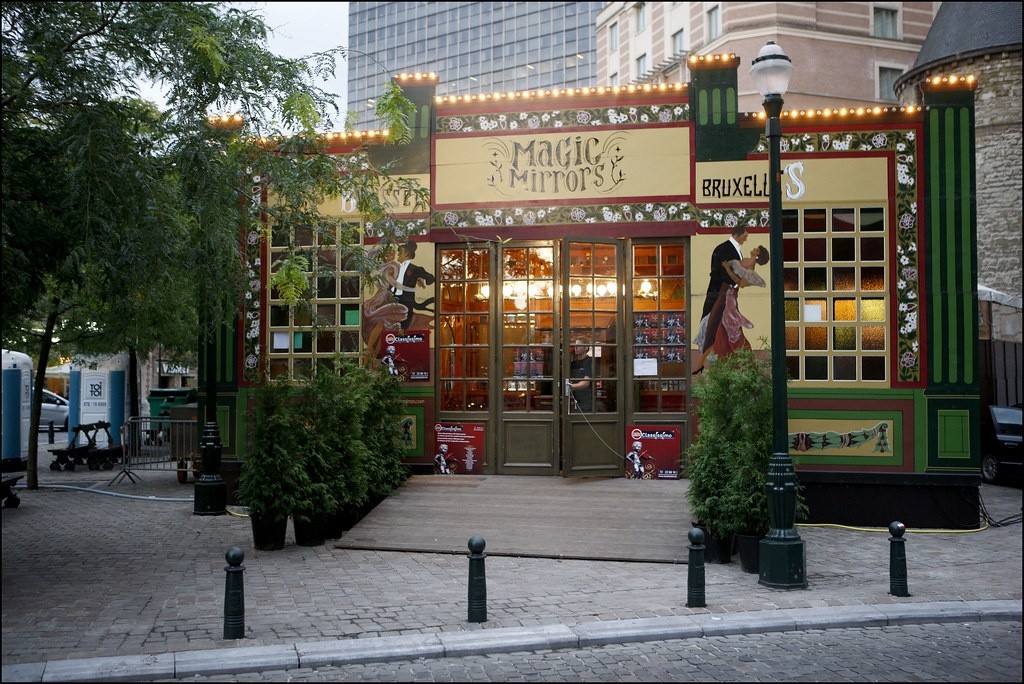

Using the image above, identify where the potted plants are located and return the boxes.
[235,350,415,551]
[726,358,811,576]
[679,350,741,563]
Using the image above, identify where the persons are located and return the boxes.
[567,335,596,413]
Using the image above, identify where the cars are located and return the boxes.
[980,404,1023,488]
[33,386,69,431]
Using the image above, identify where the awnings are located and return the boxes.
[976,282,1023,312]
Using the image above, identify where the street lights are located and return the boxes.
[745,39,809,590]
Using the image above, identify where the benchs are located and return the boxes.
[1,468,24,512]
[46,420,103,472]
[79,421,127,472]
